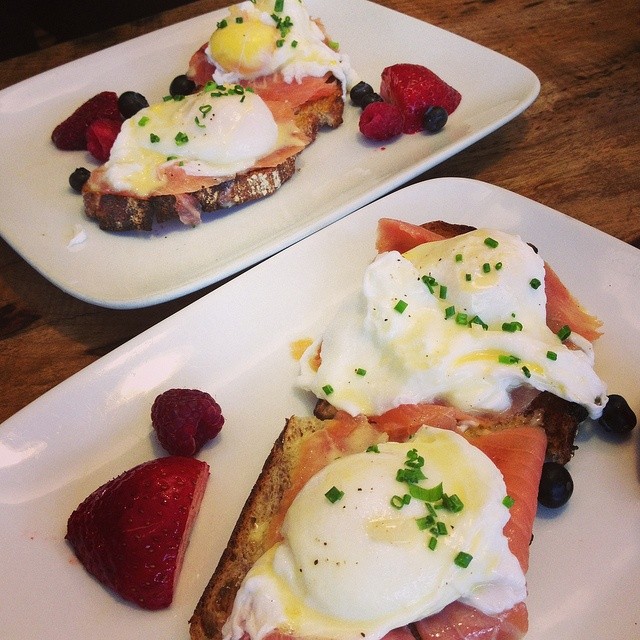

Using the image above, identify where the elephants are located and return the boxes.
[67,456,211,610]
[380,63,462,135]
[51,91,121,151]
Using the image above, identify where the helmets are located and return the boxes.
[0,0,540,310]
[1,176,640,637]
